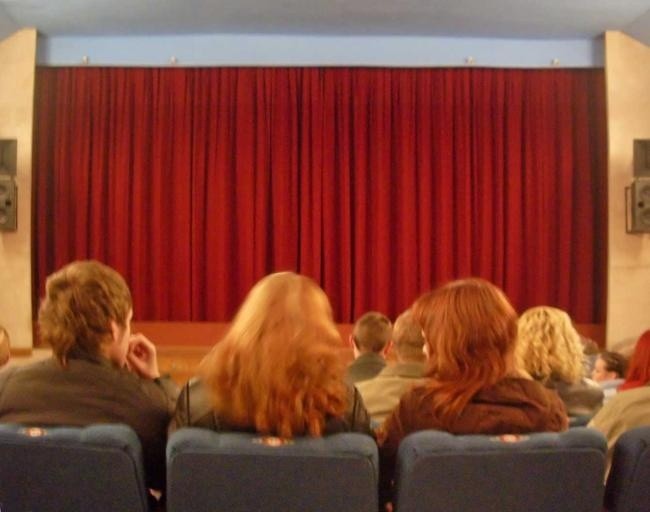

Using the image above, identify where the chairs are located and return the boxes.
[606,426,650,508]
[392,427,609,508]
[1,424,151,507]
[165,425,381,508]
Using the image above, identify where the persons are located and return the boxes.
[166,270,371,435]
[585,383,648,484]
[2,259,179,457]
[515,308,604,421]
[371,277,568,473]
[579,331,650,387]
[345,313,394,385]
[354,308,426,421]
